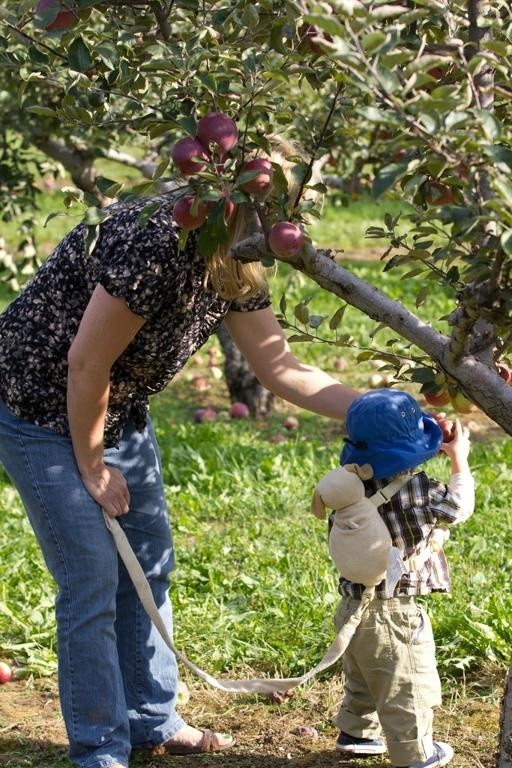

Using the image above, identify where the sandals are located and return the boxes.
[137,729,237,756]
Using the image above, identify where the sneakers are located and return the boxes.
[407,742,454,768]
[336,730,386,754]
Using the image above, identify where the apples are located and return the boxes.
[172,0,512,428]
[270,433,287,443]
[429,412,455,443]
[0,662,12,684]
[36,0,77,31]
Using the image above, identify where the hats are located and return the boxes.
[340,388,443,480]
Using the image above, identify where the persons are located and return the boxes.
[1,133,365,767]
[309,386,476,767]
